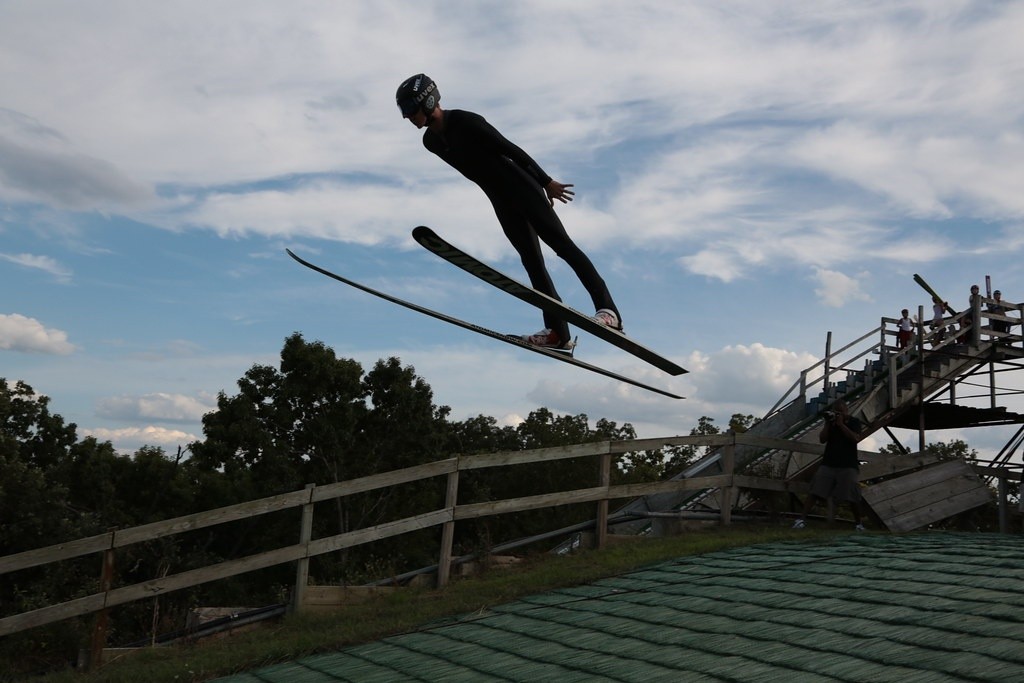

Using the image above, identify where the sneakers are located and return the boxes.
[528,328,572,350]
[593,309,623,330]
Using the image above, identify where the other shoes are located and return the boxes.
[856,524,865,531]
[791,519,808,529]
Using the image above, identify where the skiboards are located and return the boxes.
[285,226,690,400]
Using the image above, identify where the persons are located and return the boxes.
[792,399,868,534]
[897,284,1013,355]
[392,72,626,352]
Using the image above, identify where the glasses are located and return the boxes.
[402,102,421,118]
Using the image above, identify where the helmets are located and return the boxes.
[396,74,440,114]
